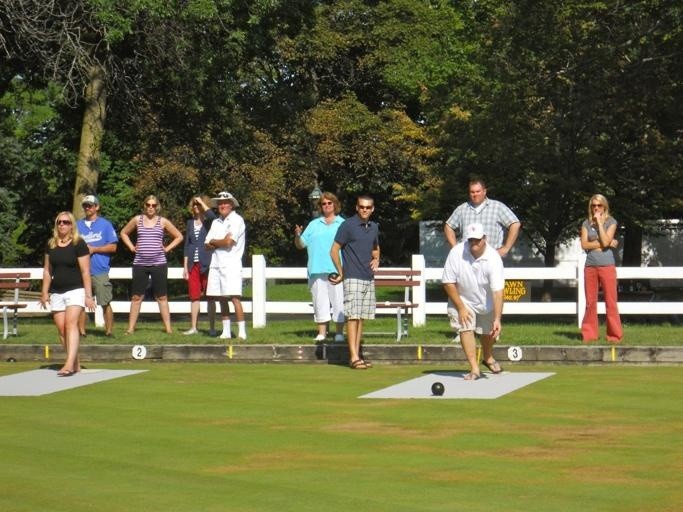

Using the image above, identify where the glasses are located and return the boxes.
[146,203,157,208]
[359,206,372,209]
[57,220,72,225]
[591,204,603,209]
[322,202,332,205]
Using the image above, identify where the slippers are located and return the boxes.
[464,373,479,380]
[482,360,502,373]
[57,370,77,376]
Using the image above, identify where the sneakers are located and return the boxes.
[209,330,217,336]
[218,334,247,342]
[183,328,198,335]
[335,334,345,342]
[104,333,115,338]
[313,334,326,341]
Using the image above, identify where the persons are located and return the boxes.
[39,210,95,378]
[180,191,217,338]
[578,192,622,343]
[204,192,247,340]
[120,194,183,336]
[329,193,382,371]
[75,194,118,337]
[293,190,345,343]
[442,180,520,344]
[437,223,504,379]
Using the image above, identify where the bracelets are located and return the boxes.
[493,319,501,323]
[87,295,92,298]
[375,258,380,263]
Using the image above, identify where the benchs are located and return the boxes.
[0,273,31,340]
[309,270,420,342]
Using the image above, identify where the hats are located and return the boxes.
[211,191,240,208]
[82,195,99,208]
[467,224,484,239]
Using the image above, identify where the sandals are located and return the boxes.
[349,358,372,369]
